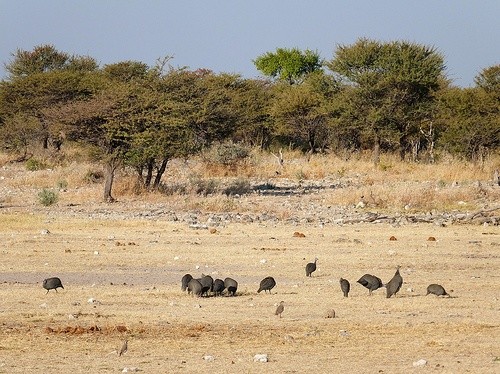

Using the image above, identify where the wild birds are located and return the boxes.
[274,300,285,318]
[356,273,383,296]
[42,276,65,295]
[385,265,403,298]
[181,272,239,299]
[118,339,129,357]
[424,283,450,298]
[338,277,351,298]
[305,257,319,277]
[256,276,276,294]
